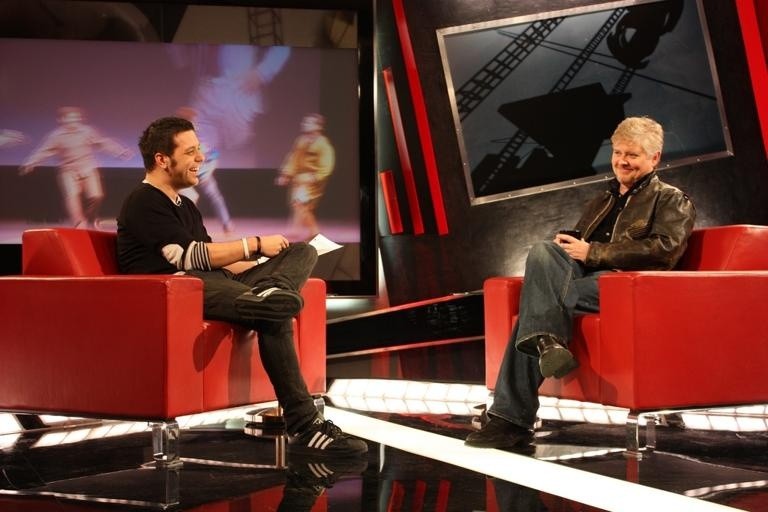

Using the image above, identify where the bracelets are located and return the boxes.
[241,238,250,262]
[256,234,263,254]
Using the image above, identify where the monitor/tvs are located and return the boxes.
[0,0,378,298]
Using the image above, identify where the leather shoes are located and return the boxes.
[464,417,536,446]
[536,336,579,381]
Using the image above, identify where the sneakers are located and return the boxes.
[231,283,305,324]
[283,461,369,496]
[284,411,369,466]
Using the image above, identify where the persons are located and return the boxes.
[464,115,694,456]
[117,119,370,458]
[277,114,335,240]
[174,108,236,239]
[19,105,134,229]
[274,457,360,510]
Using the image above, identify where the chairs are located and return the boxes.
[0,227,327,466]
[481,224,768,459]
